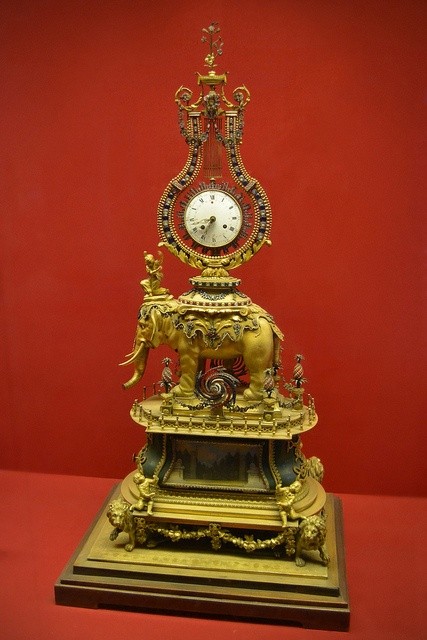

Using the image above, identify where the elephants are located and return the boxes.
[119,299,283,401]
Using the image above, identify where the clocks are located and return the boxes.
[177,181,254,258]
